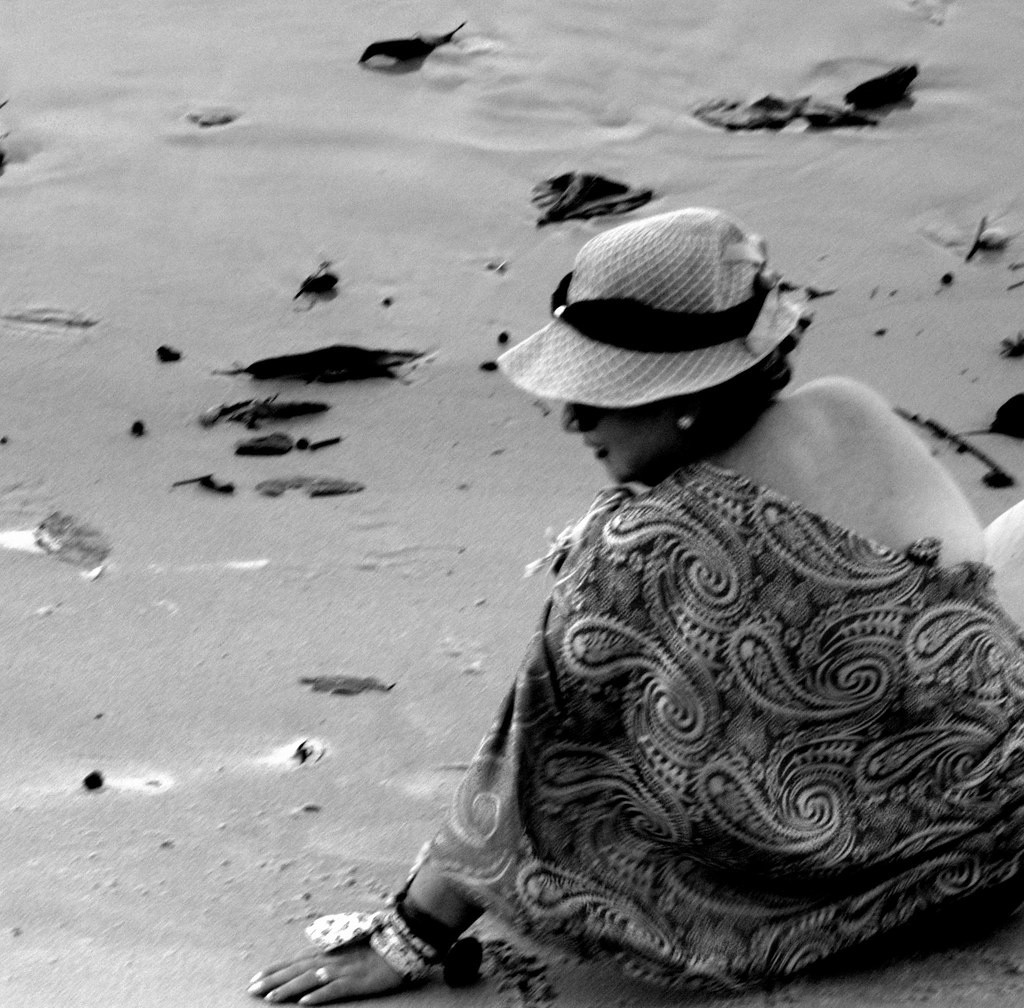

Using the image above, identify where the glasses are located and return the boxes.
[565,404,602,434]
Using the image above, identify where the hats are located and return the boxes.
[496,207,809,409]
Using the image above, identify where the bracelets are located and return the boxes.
[369,903,435,980]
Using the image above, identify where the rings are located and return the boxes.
[316,967,330,984]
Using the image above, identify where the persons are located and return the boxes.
[249,206,1024,1005]
